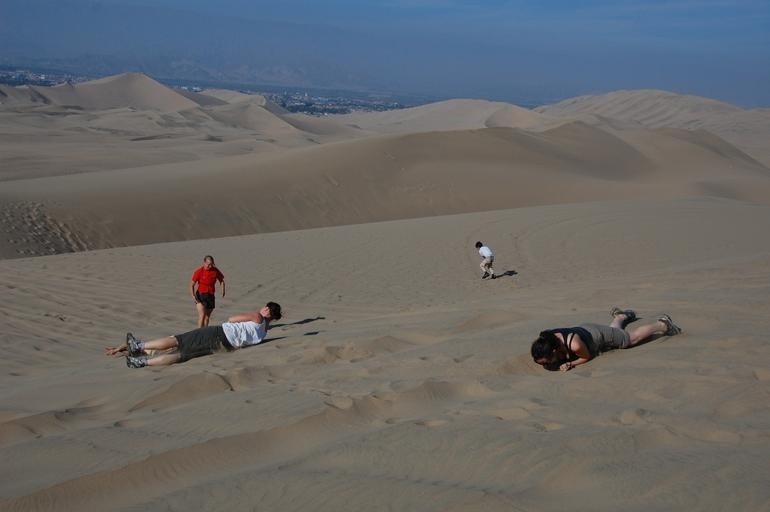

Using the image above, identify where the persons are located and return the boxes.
[531,306,681,372]
[190,255,225,328]
[105,341,130,358]
[475,241,496,279]
[124,300,284,369]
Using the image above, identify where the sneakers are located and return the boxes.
[659,313,681,335]
[482,273,497,279]
[126,332,142,356]
[125,356,145,369]
[610,306,636,323]
